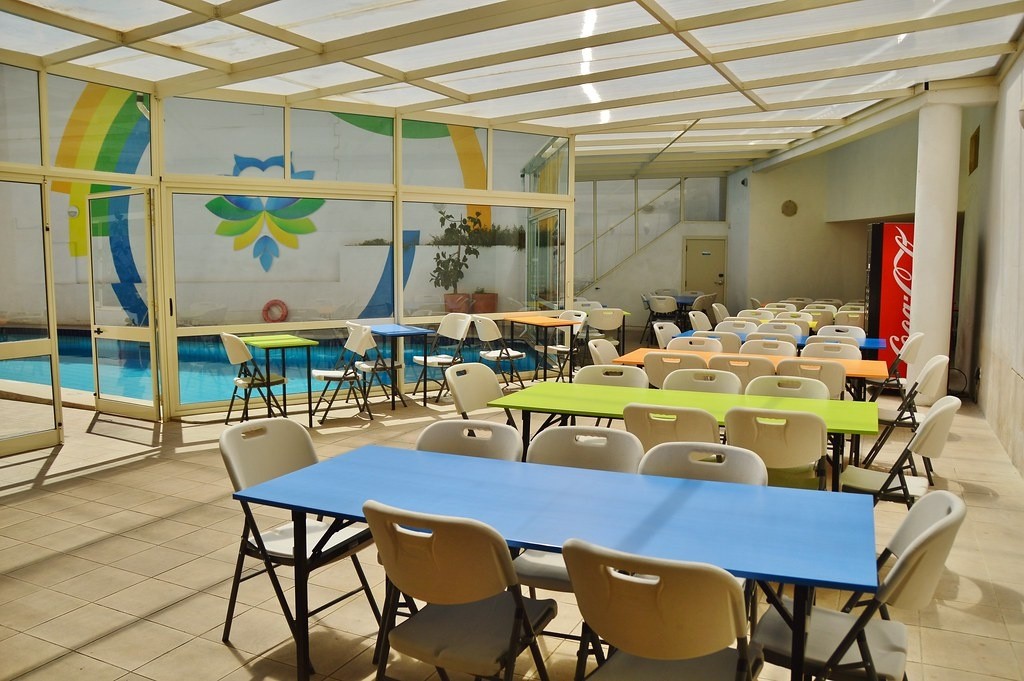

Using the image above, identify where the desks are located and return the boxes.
[368,323,437,411]
[505,315,582,383]
[737,319,818,335]
[233,444,879,681]
[486,381,876,493]
[575,309,631,365]
[559,305,607,344]
[241,333,320,428]
[642,296,697,334]
[672,329,888,401]
[613,348,889,470]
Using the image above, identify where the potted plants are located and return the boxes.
[429,210,482,315]
[472,287,498,313]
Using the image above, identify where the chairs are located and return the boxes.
[208,289,969,681]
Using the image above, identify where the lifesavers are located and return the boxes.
[261,299,288,322]
